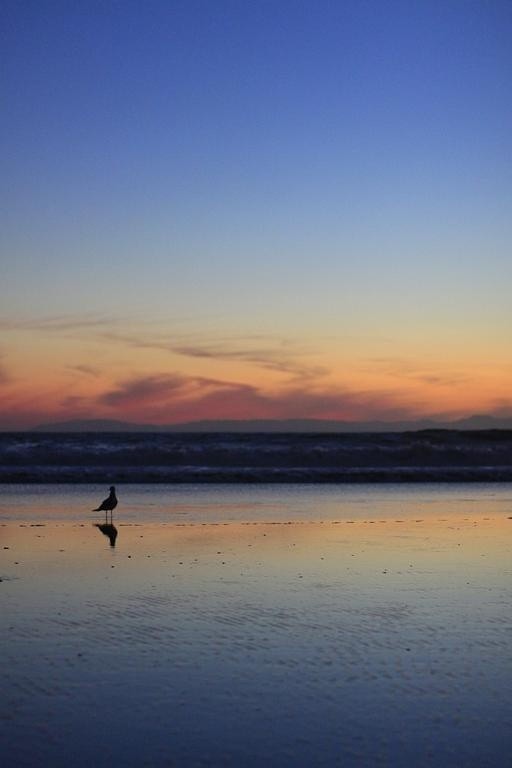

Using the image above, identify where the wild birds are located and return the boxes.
[94,525,118,547]
[92,485,118,525]
